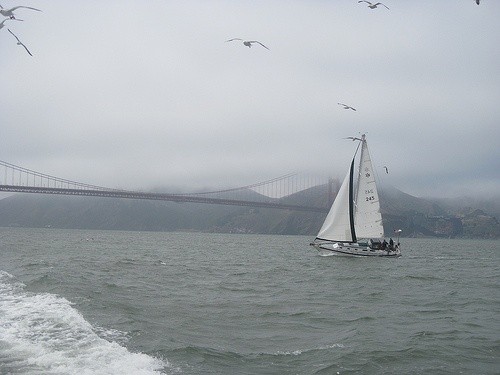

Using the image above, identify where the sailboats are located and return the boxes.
[308,133,401,257]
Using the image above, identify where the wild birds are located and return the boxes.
[0,19,24,29]
[357,0,390,10]
[0,5,44,18]
[337,102,355,111]
[224,37,270,50]
[8,29,32,56]
[342,136,365,142]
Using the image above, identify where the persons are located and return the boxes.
[383,237,393,247]
